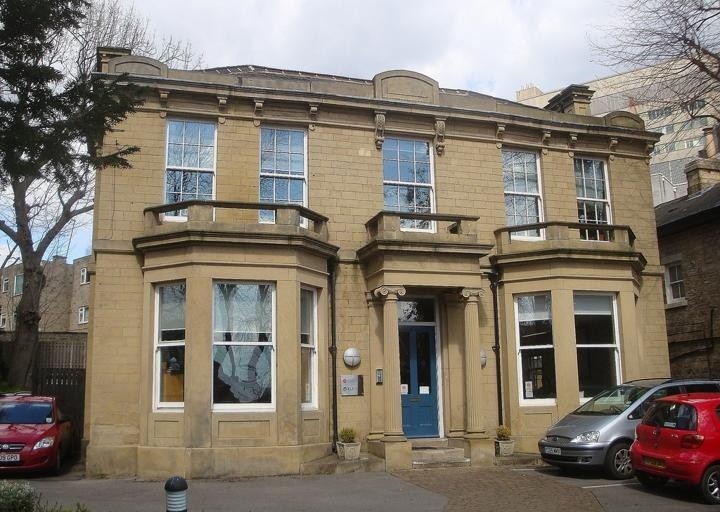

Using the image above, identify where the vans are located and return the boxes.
[540,376,719,482]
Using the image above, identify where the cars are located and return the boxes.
[629,392,719,503]
[0,390,73,477]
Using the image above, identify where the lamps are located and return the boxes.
[344,347,361,368]
[480,349,487,368]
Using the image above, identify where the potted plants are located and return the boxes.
[491,421,518,458]
[334,424,365,461]
